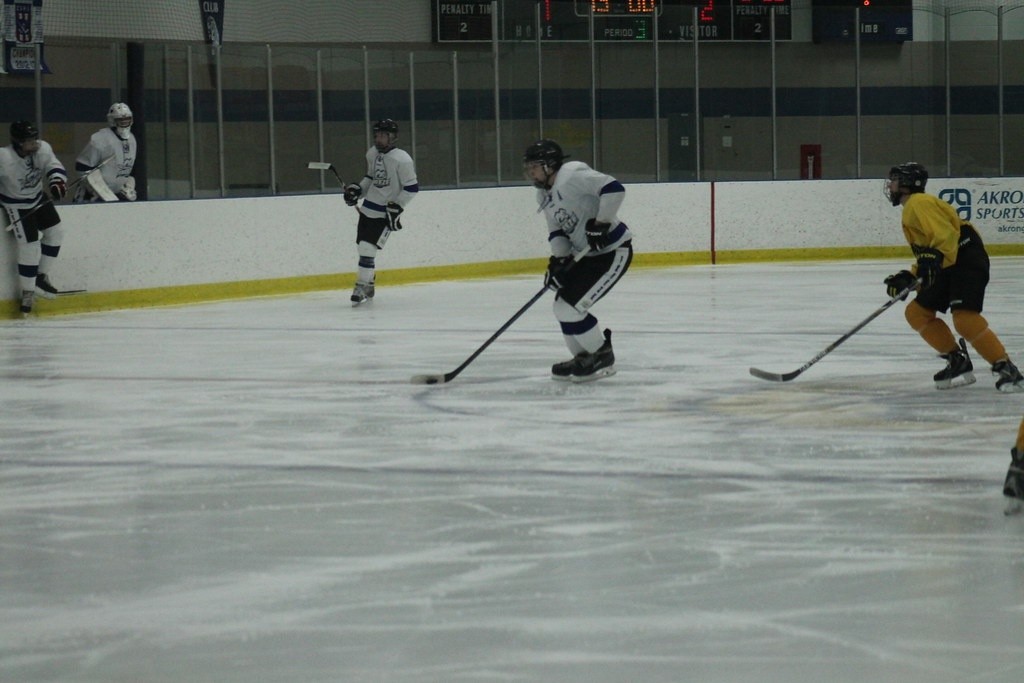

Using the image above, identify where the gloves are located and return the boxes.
[584,218,611,252]
[343,182,362,206]
[543,256,569,290]
[385,202,403,231]
[49,177,67,201]
[883,270,915,302]
[915,245,945,289]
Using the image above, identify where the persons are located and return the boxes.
[74,102,137,204]
[524,138,633,383]
[884,162,1024,393]
[1004,416,1024,516]
[0,120,66,319]
[344,119,418,307]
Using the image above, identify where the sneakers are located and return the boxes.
[990,360,1024,391]
[351,274,376,308]
[34,273,58,301]
[20,289,35,318]
[933,337,976,390]
[551,328,615,383]
[1002,447,1024,514]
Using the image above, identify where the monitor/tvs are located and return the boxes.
[811,0,914,41]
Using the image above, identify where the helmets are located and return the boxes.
[883,162,929,206]
[372,119,399,154]
[519,139,562,190]
[107,102,133,140]
[10,119,41,158]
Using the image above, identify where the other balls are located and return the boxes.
[426,378,438,384]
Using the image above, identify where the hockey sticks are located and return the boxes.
[746,236,971,383]
[303,161,371,241]
[3,153,116,233]
[408,243,596,386]
